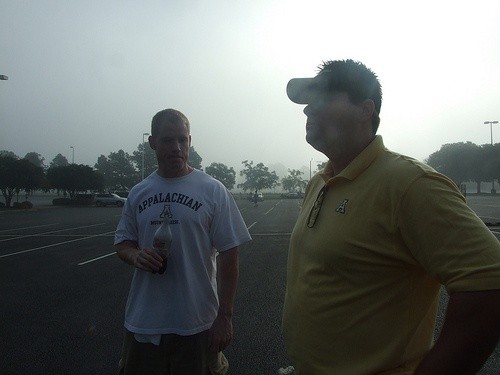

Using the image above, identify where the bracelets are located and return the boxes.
[218,312,232,316]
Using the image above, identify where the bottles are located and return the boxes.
[149,215,173,275]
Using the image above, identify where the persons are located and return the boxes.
[113,109,253,375]
[280,60,500,375]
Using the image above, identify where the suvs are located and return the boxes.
[94,192,127,207]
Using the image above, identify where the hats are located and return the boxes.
[286,73,380,113]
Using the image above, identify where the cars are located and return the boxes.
[251,193,264,202]
[288,192,298,199]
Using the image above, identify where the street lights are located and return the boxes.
[142,133,150,181]
[310,157,313,180]
[484,121,499,197]
[70,146,74,163]
